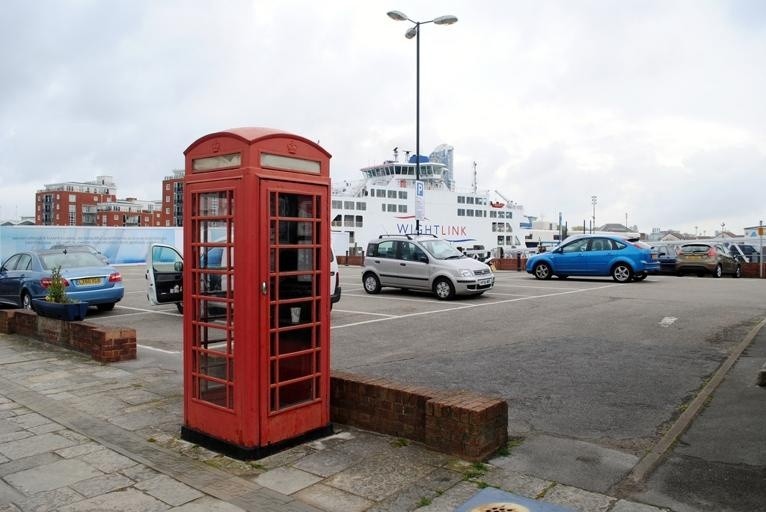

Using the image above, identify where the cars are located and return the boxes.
[0,246,124,311]
[525,234,661,282]
[675,242,743,278]
[649,245,676,275]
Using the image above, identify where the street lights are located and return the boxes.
[387,11,458,236]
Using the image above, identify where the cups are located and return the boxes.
[291,307,301,323]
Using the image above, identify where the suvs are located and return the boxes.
[361,234,495,301]
[145,235,341,321]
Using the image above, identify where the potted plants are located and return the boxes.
[32,267,89,321]
